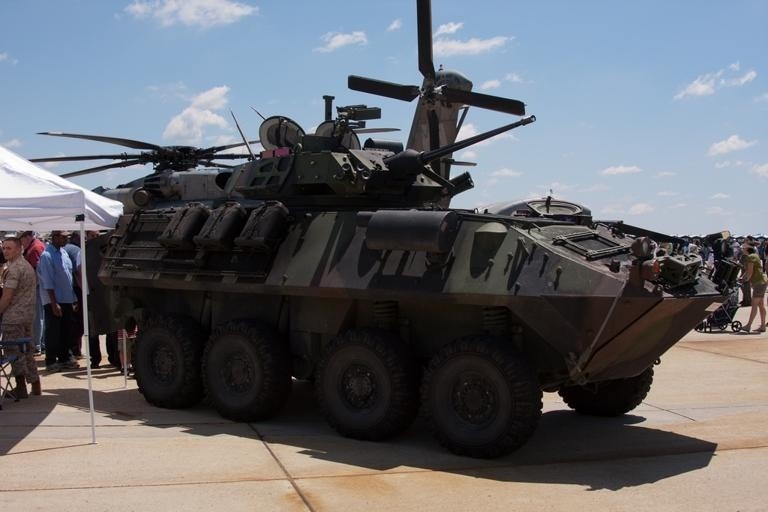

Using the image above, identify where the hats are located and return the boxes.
[16,231,32,238]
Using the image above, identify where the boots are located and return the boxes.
[3,375,41,399]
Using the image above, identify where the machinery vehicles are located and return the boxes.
[95,114,729,458]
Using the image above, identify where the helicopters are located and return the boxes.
[25,0,526,210]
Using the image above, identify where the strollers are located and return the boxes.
[695,283,744,332]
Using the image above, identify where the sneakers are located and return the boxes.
[33,351,46,356]
[90,355,122,369]
[45,360,81,372]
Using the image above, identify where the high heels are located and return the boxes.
[739,325,766,333]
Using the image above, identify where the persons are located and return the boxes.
[661,233,768,332]
[0,231,143,400]
